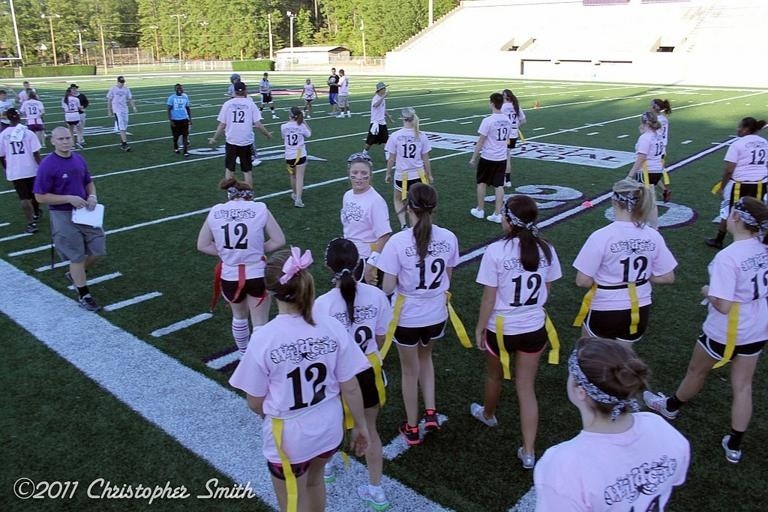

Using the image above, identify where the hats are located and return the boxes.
[376,82,389,92]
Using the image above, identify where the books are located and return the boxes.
[71,204,104,229]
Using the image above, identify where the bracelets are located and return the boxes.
[88,194,96,197]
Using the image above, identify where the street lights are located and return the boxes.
[166,13,188,71]
[72,28,87,56]
[3,0,23,59]
[286,10,298,71]
[41,13,62,67]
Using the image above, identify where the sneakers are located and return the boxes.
[471,403,497,427]
[663,190,671,203]
[291,193,304,207]
[722,435,741,463]
[487,212,502,223]
[518,447,535,469]
[78,294,101,311]
[643,390,679,419]
[705,238,722,248]
[27,209,42,232]
[323,464,335,482]
[358,485,389,510]
[121,144,130,151]
[471,208,484,218]
[399,413,440,444]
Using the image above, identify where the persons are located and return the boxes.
[0,80,90,150]
[165,82,192,157]
[196,66,767,511]
[31,126,107,313]
[0,107,42,234]
[105,75,137,152]
